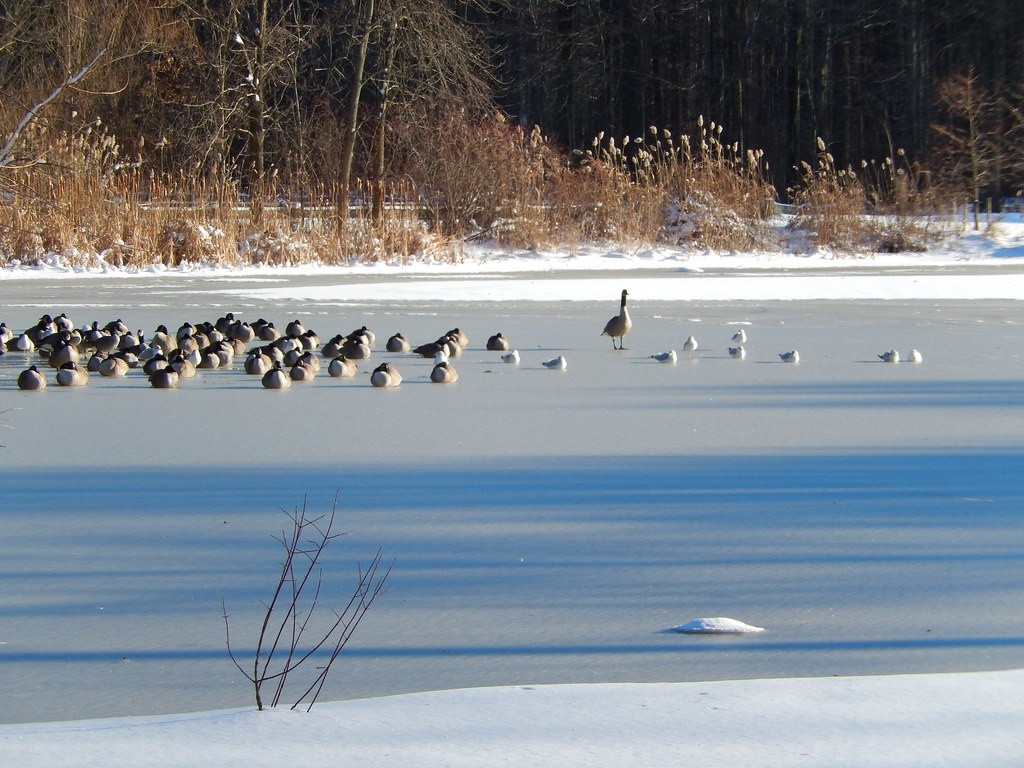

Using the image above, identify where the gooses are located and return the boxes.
[778,351,799,363]
[0,312,470,389]
[500,350,521,364]
[682,336,698,351]
[731,329,747,346]
[541,355,567,370]
[907,349,922,362]
[599,289,633,351]
[877,350,900,363]
[728,346,746,358]
[648,350,677,364]
[486,333,509,350]
